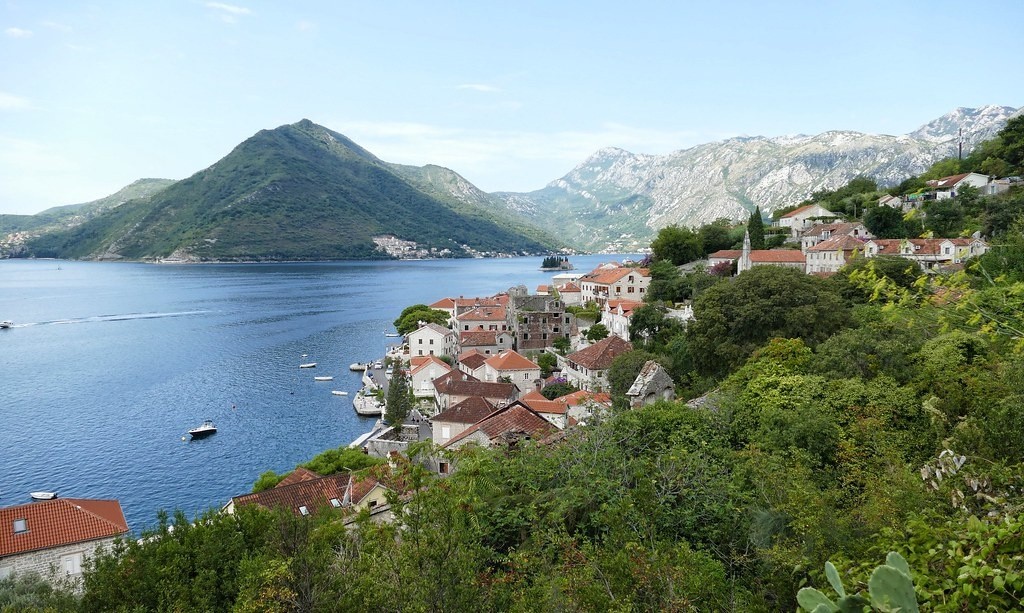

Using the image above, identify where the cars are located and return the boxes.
[375,362,383,369]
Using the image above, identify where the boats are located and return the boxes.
[331,390,348,396]
[0,321,15,328]
[29,491,58,500]
[314,376,332,381]
[385,333,401,337]
[188,419,217,437]
[299,362,318,368]
